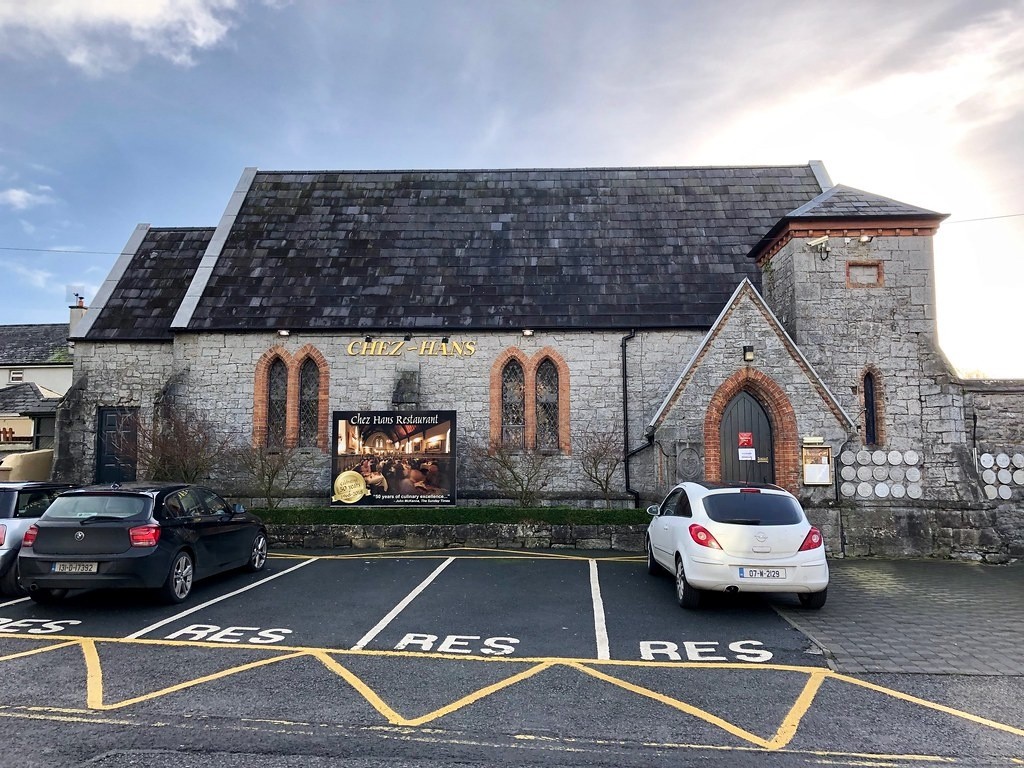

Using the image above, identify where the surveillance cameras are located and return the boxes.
[807,235,828,247]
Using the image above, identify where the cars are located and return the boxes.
[17,479,270,612]
[645,478,830,610]
[0,479,86,599]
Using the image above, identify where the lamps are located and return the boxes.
[744,346,754,361]
[365,335,372,342]
[278,329,291,336]
[404,334,411,341]
[858,235,873,246]
[522,330,534,336]
[442,337,448,343]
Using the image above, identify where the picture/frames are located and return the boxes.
[426,440,442,452]
[349,430,357,450]
[412,440,422,452]
